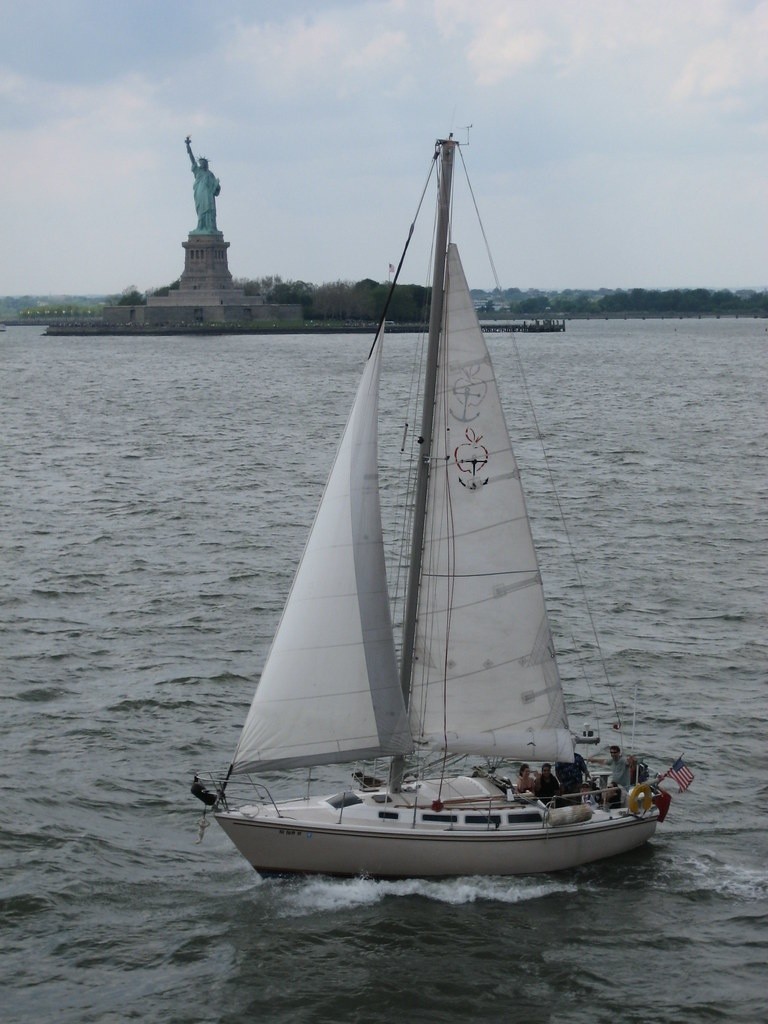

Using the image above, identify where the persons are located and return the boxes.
[516,746,636,809]
[184,136,223,235]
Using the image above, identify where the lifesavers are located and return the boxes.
[630,784,652,811]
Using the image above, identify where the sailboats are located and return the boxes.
[190,140,671,880]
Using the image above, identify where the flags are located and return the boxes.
[665,763,695,794]
